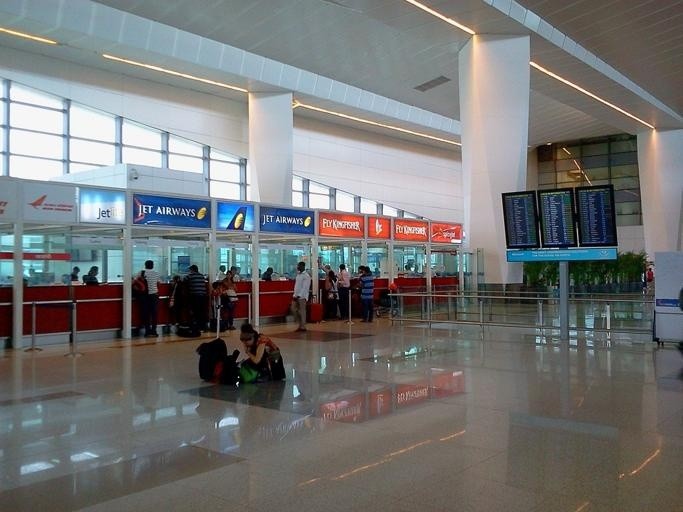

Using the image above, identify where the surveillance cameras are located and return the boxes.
[132,172,138,179]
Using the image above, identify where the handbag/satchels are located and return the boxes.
[327,279,339,301]
[196,338,243,386]
[168,296,175,308]
[265,350,286,381]
[178,328,201,337]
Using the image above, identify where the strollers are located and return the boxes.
[375,290,397,318]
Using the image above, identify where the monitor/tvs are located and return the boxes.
[502,190,540,249]
[537,188,577,248]
[575,185,617,246]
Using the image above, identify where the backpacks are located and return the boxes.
[133,271,148,298]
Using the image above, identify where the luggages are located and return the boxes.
[307,295,324,323]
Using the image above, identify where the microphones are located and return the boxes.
[117,275,124,277]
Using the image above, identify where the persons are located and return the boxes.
[88,266,108,286]
[137,260,160,338]
[262,268,273,282]
[319,260,374,323]
[240,324,283,383]
[290,262,312,331]
[70,267,80,280]
[170,265,241,332]
[647,268,653,281]
